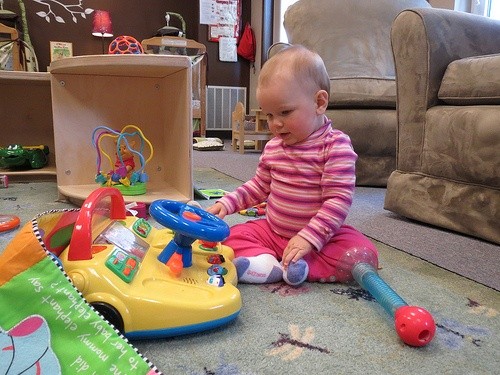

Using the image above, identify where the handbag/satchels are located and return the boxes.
[237,22,256,63]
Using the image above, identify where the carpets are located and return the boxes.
[193,136,500,292]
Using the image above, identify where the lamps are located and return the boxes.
[92,10,114,55]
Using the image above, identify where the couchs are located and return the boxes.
[267,0,500,244]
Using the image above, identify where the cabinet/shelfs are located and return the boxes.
[231,103,274,153]
[0,55,193,209]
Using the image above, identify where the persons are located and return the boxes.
[205,45,380,286]
